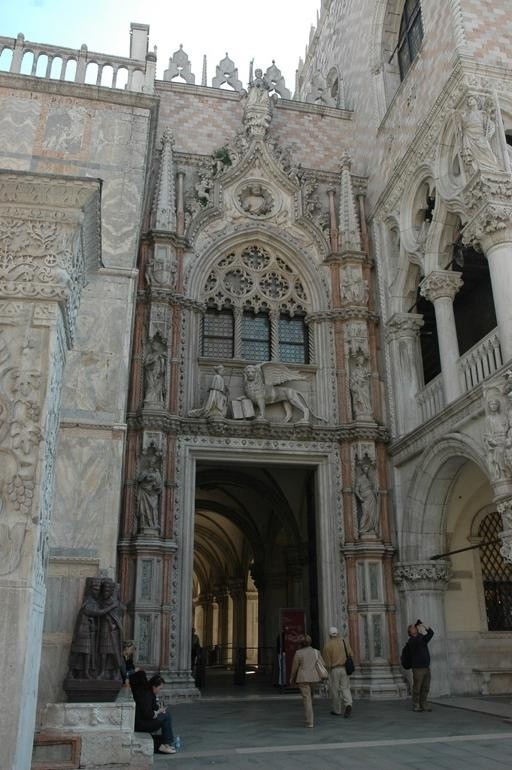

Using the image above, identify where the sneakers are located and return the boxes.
[158,744,177,754]
[413,708,433,712]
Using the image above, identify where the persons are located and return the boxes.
[134,675,177,754]
[185,362,232,419]
[195,178,215,203]
[339,276,353,300]
[144,257,157,287]
[402,619,436,713]
[305,193,323,214]
[450,92,502,179]
[320,625,355,718]
[355,462,387,537]
[289,632,327,729]
[70,579,121,679]
[135,455,164,531]
[479,395,511,482]
[243,183,269,216]
[362,279,372,305]
[97,576,126,680]
[348,355,373,419]
[171,258,181,291]
[241,66,272,109]
[143,340,169,404]
[191,627,202,673]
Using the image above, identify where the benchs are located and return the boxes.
[133,731,154,765]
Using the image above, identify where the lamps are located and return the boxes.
[424,196,432,223]
[453,243,483,267]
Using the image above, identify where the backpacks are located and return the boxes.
[344,655,355,675]
[400,646,412,669]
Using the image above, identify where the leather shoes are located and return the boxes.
[330,712,341,716]
[344,705,352,717]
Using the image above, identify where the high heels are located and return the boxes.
[304,720,314,729]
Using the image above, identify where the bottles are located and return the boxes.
[175,736,183,753]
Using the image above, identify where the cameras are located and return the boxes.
[415,619,422,627]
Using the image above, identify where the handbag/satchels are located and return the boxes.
[316,662,329,680]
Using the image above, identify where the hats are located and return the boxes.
[328,626,337,637]
